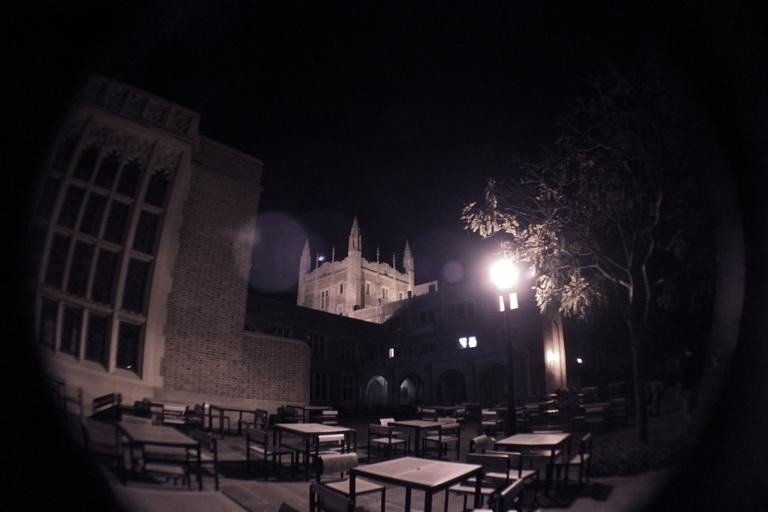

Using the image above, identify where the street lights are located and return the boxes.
[490,256,524,439]
